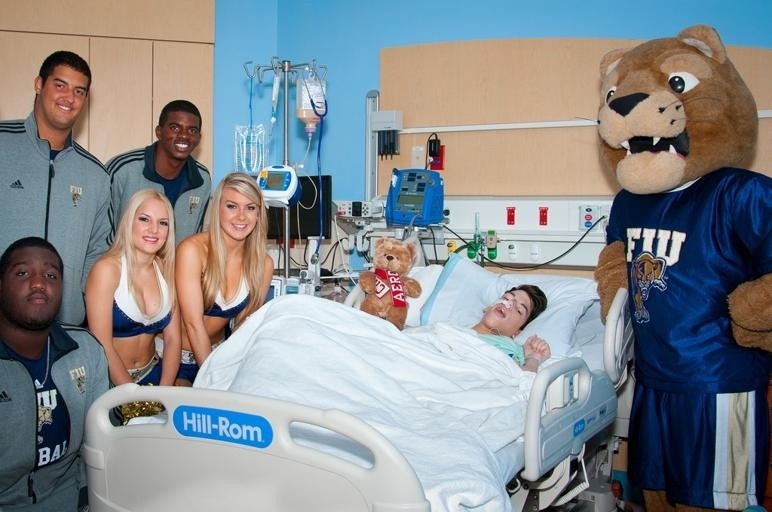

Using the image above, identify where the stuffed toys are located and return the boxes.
[358,238,421,330]
[593,27,772,511]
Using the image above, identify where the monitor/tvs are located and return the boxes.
[250,175,331,240]
[265,285,275,304]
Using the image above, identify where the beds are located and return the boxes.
[79,263,638,511]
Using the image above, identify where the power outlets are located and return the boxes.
[600,207,611,229]
[427,143,445,171]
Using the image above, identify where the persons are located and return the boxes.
[1,52,111,327]
[155,173,273,386]
[0,237,108,512]
[470,285,551,372]
[103,100,211,249]
[88,189,180,386]
